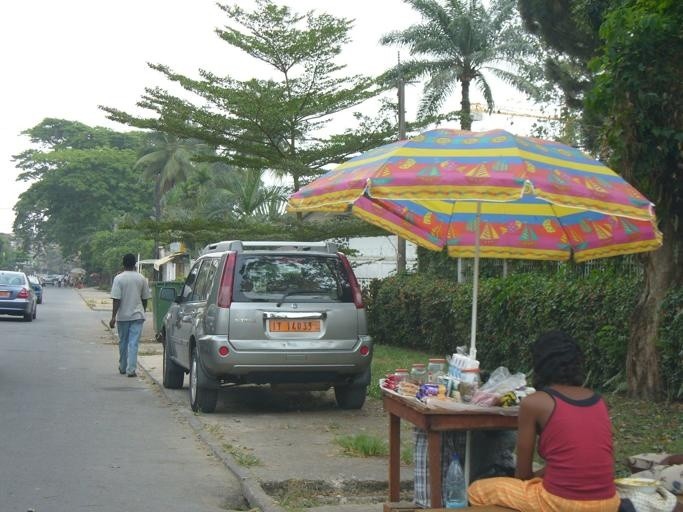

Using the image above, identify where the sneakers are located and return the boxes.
[118,366,137,377]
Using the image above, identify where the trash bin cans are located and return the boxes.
[152,281,185,342]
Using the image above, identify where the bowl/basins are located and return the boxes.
[613,477,663,494]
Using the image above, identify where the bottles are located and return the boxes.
[393,359,447,389]
[443,455,470,512]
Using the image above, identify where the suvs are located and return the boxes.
[156,238,376,413]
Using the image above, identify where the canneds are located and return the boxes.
[428,359,446,382]
[410,364,426,382]
[395,369,410,382]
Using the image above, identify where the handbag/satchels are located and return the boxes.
[412,425,521,510]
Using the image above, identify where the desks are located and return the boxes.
[379,384,539,512]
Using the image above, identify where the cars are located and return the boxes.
[0,271,42,321]
[43,273,64,288]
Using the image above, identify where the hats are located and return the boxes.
[123,253,136,266]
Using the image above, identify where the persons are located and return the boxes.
[108,253,150,377]
[33,267,88,289]
[283,125,663,490]
[466,331,620,511]
